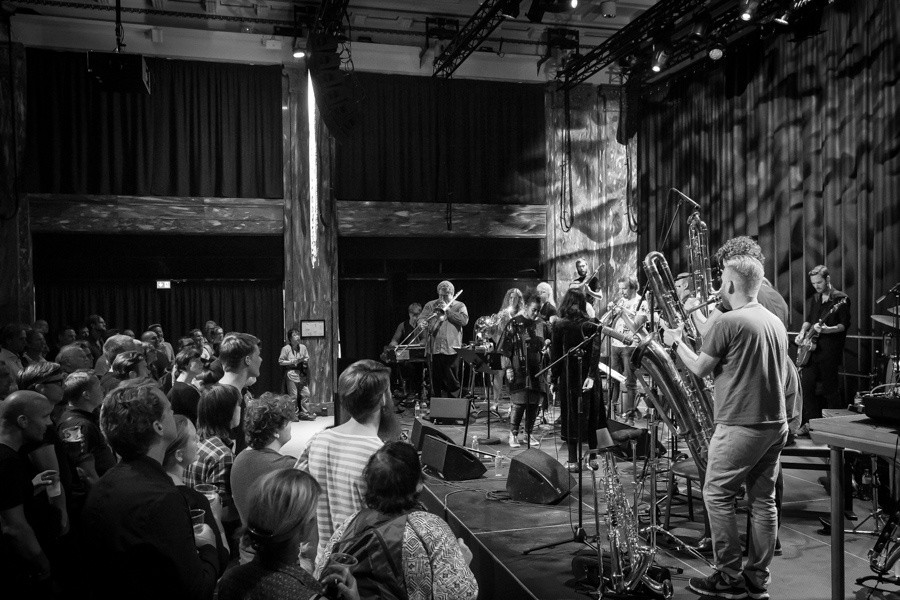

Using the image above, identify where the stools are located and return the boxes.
[664,458,701,530]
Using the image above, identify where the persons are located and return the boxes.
[0,281,479,600]
[493,236,850,600]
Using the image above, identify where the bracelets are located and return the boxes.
[671,340,682,351]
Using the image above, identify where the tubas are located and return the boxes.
[685,214,722,323]
[600,252,726,479]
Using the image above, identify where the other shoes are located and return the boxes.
[563,461,579,473]
[584,460,600,471]
[798,423,812,436]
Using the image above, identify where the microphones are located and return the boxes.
[427,313,437,322]
[597,323,634,344]
[672,188,701,210]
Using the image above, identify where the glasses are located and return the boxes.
[43,379,65,388]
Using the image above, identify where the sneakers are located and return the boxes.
[748,588,769,600]
[688,571,747,598]
[523,433,539,445]
[508,436,521,448]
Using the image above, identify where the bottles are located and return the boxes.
[495,451,502,477]
[415,401,420,419]
[472,436,480,458]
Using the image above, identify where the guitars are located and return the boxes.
[797,296,849,366]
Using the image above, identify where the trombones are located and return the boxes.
[394,288,464,356]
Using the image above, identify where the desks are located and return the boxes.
[809,413,900,600]
[822,409,856,418]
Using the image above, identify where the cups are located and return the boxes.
[190,509,206,535]
[63,425,82,442]
[41,471,61,498]
[326,552,359,600]
[193,483,218,504]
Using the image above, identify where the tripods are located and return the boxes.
[853,454,888,533]
[522,331,719,576]
[475,353,505,425]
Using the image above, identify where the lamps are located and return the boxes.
[293,37,307,58]
[600,1,616,18]
[651,0,791,72]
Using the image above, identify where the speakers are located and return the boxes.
[430,397,469,426]
[506,447,577,505]
[410,417,456,452]
[89,54,152,95]
[307,33,361,136]
[421,434,487,481]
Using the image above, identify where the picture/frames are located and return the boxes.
[300,320,325,338]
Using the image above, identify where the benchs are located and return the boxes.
[780,438,862,520]
